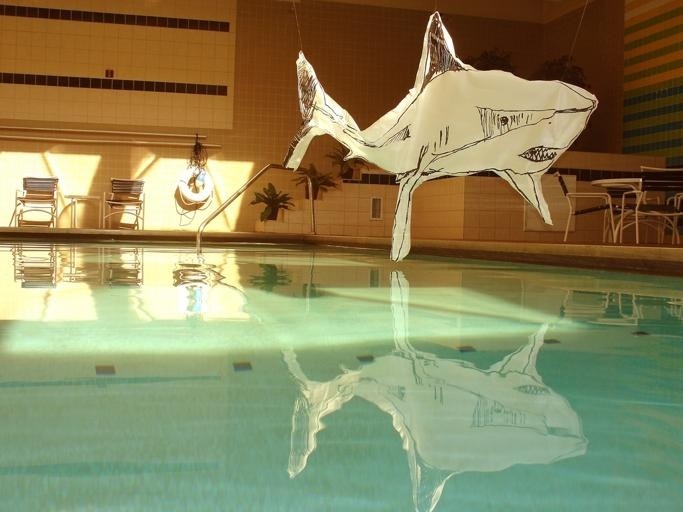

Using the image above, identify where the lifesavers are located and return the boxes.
[178,168,213,201]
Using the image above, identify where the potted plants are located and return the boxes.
[248,262,291,294]
[250,184,292,220]
[294,162,334,197]
[327,142,366,181]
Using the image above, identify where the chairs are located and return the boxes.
[7,176,58,228]
[7,242,141,290]
[551,164,682,244]
[556,289,682,328]
[101,179,142,231]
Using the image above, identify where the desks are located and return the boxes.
[64,195,103,227]
